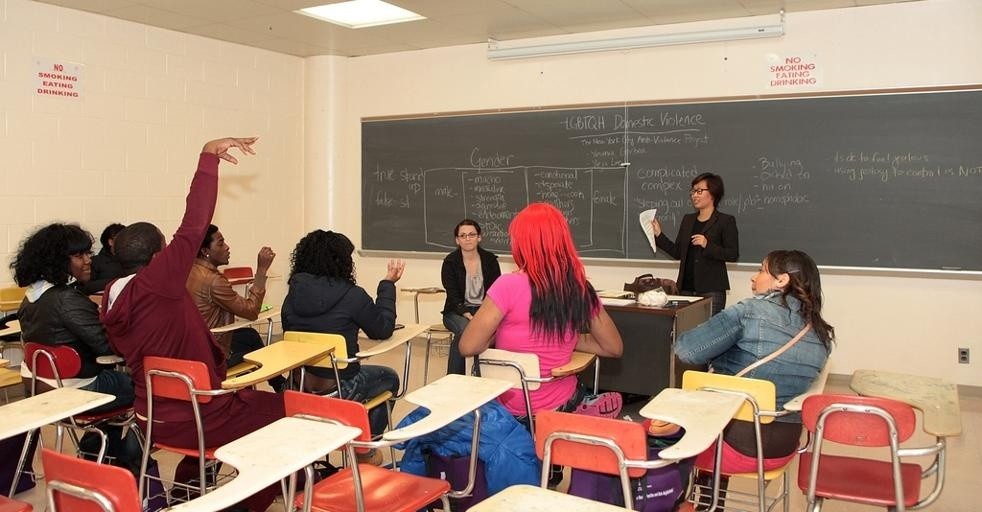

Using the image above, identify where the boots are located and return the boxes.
[694,470,728,512]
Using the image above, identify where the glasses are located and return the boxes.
[458,233,477,239]
[689,186,710,195]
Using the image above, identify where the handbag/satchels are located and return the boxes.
[422,448,486,512]
[624,274,679,295]
[564,446,687,511]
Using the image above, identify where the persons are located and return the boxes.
[186,224,288,392]
[279,227,406,470]
[649,173,738,318]
[9,222,153,475]
[441,219,501,383]
[100,137,287,512]
[458,199,625,459]
[666,251,832,512]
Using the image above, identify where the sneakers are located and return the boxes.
[570,392,623,419]
[357,449,382,466]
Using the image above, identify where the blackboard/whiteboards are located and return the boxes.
[358,84,982,279]
